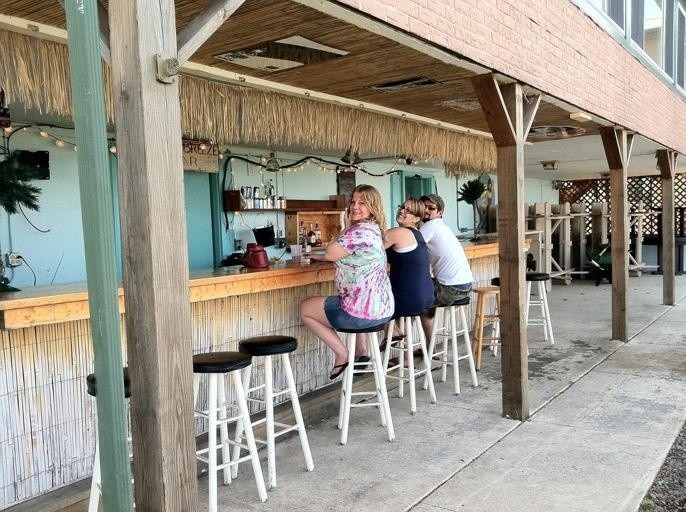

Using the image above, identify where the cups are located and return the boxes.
[301,245,311,266]
[290,245,302,263]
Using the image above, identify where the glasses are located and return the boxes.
[397,204,413,213]
[424,203,440,210]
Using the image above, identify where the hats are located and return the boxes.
[419,194,445,211]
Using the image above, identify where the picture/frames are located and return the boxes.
[337,165,356,209]
[477,171,495,213]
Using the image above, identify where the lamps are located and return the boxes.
[0,87,11,128]
[265,152,280,172]
[341,146,363,165]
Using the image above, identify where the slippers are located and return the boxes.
[391,335,406,347]
[379,336,402,353]
[353,355,370,376]
[414,349,440,361]
[329,362,349,380]
[388,358,416,369]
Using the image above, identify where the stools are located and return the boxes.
[423,296,478,395]
[193,352,268,512]
[336,323,396,445]
[383,313,437,415]
[473,287,500,371]
[490,278,530,358]
[230,335,315,491]
[86,367,137,512]
[525,272,555,345]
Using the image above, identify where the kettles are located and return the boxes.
[241,245,270,271]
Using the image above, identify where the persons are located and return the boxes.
[299,184,394,379]
[414,194,473,358]
[378,195,434,353]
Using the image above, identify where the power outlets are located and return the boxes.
[235,240,242,251]
[6,253,23,268]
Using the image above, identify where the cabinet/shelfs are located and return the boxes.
[285,211,343,244]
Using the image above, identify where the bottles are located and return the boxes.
[298,220,321,246]
[300,238,312,267]
[240,178,286,209]
